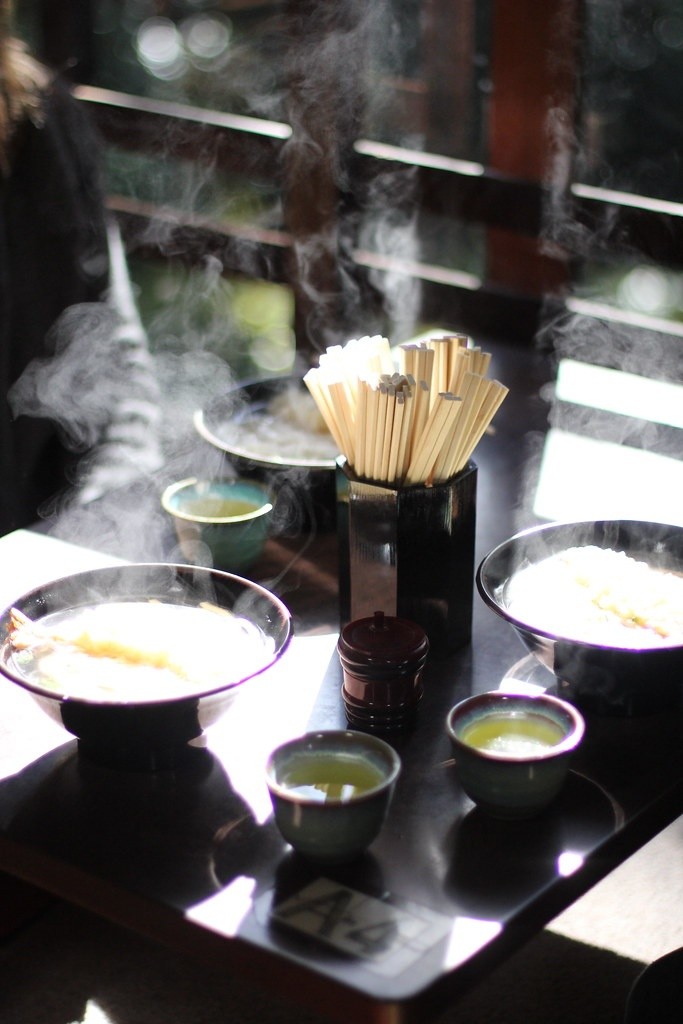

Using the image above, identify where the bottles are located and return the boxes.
[336,610,430,740]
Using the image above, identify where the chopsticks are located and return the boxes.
[302,330,510,486]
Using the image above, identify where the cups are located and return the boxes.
[446,690,586,817]
[161,475,279,571]
[265,729,403,860]
[334,455,479,747]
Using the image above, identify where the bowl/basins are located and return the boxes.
[193,376,339,549]
[0,563,293,744]
[476,520,682,703]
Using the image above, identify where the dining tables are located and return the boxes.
[0,451,683,1022]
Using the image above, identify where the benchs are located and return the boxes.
[69,84,682,464]
